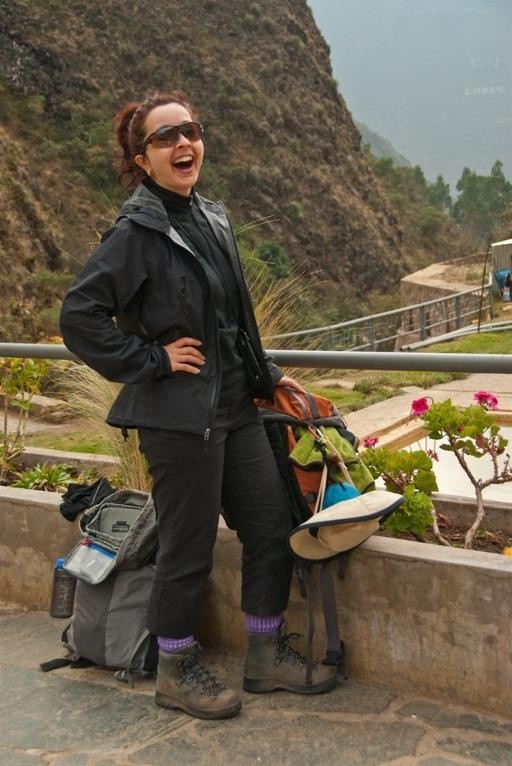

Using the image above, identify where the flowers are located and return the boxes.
[352,392,511,551]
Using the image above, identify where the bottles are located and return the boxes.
[49,559,75,618]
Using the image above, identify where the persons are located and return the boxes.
[60,90,338,720]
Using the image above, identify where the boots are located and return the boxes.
[242,618,338,696]
[154,639,242,721]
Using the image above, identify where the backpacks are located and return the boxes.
[38,488,160,691]
[254,386,377,525]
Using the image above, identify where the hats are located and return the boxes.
[287,489,406,564]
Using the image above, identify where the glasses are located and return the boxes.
[145,122,205,149]
